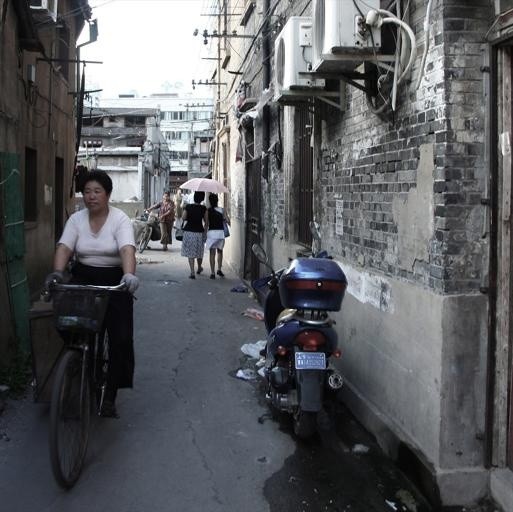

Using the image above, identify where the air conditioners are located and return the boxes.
[313,0,381,78]
[30,0,57,23]
[274,16,325,107]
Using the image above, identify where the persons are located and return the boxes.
[181,190,189,228]
[172,188,182,227]
[182,192,207,279]
[46,168,139,418]
[144,191,174,251]
[204,194,231,279]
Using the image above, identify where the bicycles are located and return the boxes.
[138,208,162,253]
[42,280,138,489]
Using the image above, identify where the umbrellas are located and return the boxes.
[182,178,230,206]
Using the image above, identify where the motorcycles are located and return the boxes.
[252,220,349,437]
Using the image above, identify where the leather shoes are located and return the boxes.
[196,268,204,274]
[188,275,196,279]
[217,270,225,277]
[101,400,116,419]
[210,274,216,279]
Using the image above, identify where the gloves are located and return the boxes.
[121,273,140,294]
[44,270,65,293]
